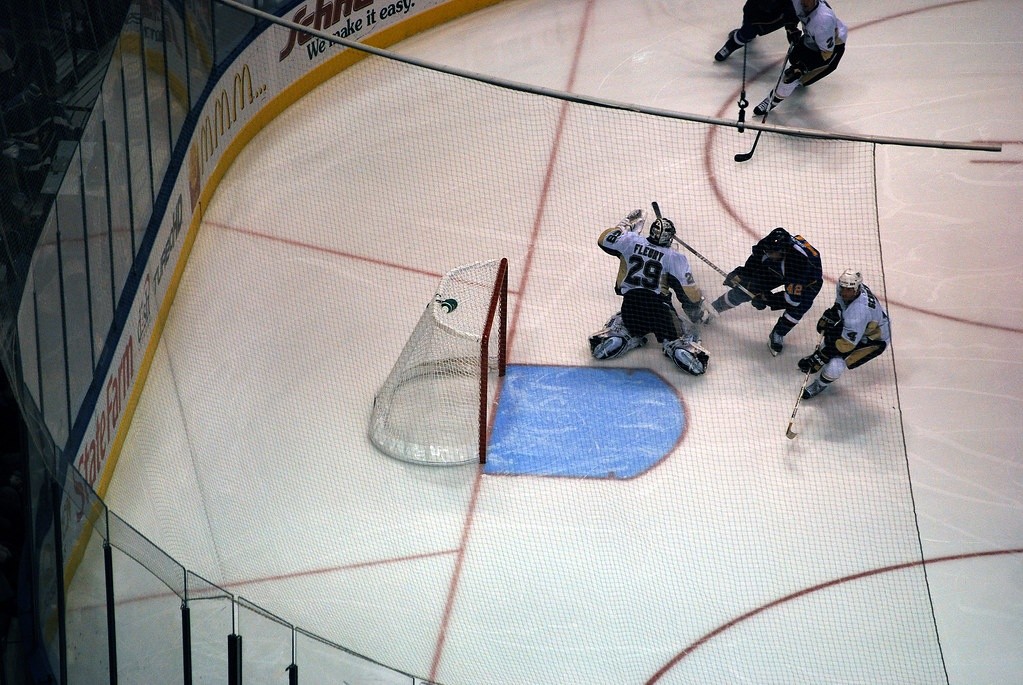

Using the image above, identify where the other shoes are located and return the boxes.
[593,337,622,359]
[676,349,703,374]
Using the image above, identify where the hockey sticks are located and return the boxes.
[733,41,794,162]
[652,201,755,299]
[785,330,824,440]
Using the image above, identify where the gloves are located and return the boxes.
[799,350,830,374]
[817,309,840,336]
[783,62,809,84]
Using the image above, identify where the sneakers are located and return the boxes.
[767,331,783,357]
[753,97,777,117]
[715,44,732,61]
[701,308,712,325]
[802,380,827,399]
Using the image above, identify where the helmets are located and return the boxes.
[839,268,863,296]
[759,228,794,262]
[650,218,675,247]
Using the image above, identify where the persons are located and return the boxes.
[705,227,822,356]
[3,43,83,229]
[715,0,847,118]
[588,209,710,375]
[0,464,24,685]
[797,269,891,398]
